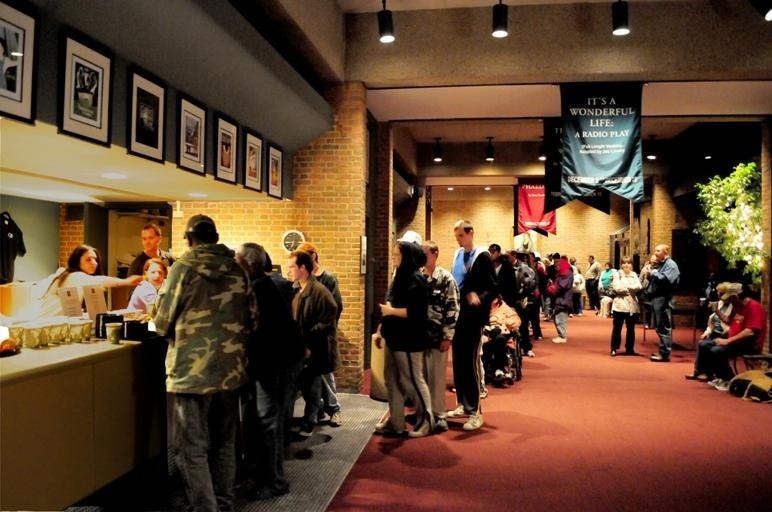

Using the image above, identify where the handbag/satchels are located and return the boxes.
[546,284,559,295]
[727,369,772,403]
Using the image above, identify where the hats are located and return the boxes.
[183,215,216,239]
[396,230,422,246]
[721,283,743,301]
[551,253,560,259]
[716,282,730,293]
[293,240,319,253]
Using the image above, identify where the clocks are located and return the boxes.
[281,229,306,254]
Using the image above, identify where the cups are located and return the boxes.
[711,302,719,311]
[105,323,123,345]
[60,322,69,342]
[700,298,706,306]
[8,324,24,348]
[72,321,83,343]
[25,325,41,348]
[48,322,61,344]
[40,324,50,346]
[82,320,93,342]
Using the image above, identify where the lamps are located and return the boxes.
[432,138,444,164]
[377,1,395,44]
[540,144,549,163]
[611,2,630,36]
[491,1,508,39]
[485,136,495,161]
[646,132,655,159]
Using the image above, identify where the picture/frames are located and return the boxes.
[177,90,208,175]
[243,126,264,192]
[55,24,114,148]
[213,111,238,185]
[266,140,284,199]
[126,68,166,164]
[0,1,39,125]
[360,235,367,275]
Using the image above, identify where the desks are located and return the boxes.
[642,300,705,349]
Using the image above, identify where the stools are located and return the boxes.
[731,353,758,376]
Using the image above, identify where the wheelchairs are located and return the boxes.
[483,332,522,381]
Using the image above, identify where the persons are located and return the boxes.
[610,256,643,357]
[646,244,681,362]
[131,223,175,278]
[638,255,659,329]
[371,240,428,439]
[708,284,767,391]
[127,257,168,313]
[232,241,343,462]
[452,220,497,431]
[156,215,252,511]
[685,282,733,382]
[489,243,617,356]
[404,240,462,431]
[66,244,142,311]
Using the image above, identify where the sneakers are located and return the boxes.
[447,405,481,417]
[375,417,404,434]
[651,355,670,362]
[685,371,697,379]
[708,379,722,386]
[495,368,504,376]
[436,415,449,430]
[300,421,314,436]
[330,413,343,426]
[697,373,714,380]
[552,337,566,344]
[463,415,484,430]
[610,350,616,356]
[407,424,431,437]
[480,389,487,398]
[715,381,729,391]
[627,351,639,356]
[652,353,661,356]
[527,350,535,357]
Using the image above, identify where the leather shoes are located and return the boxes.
[253,483,289,499]
